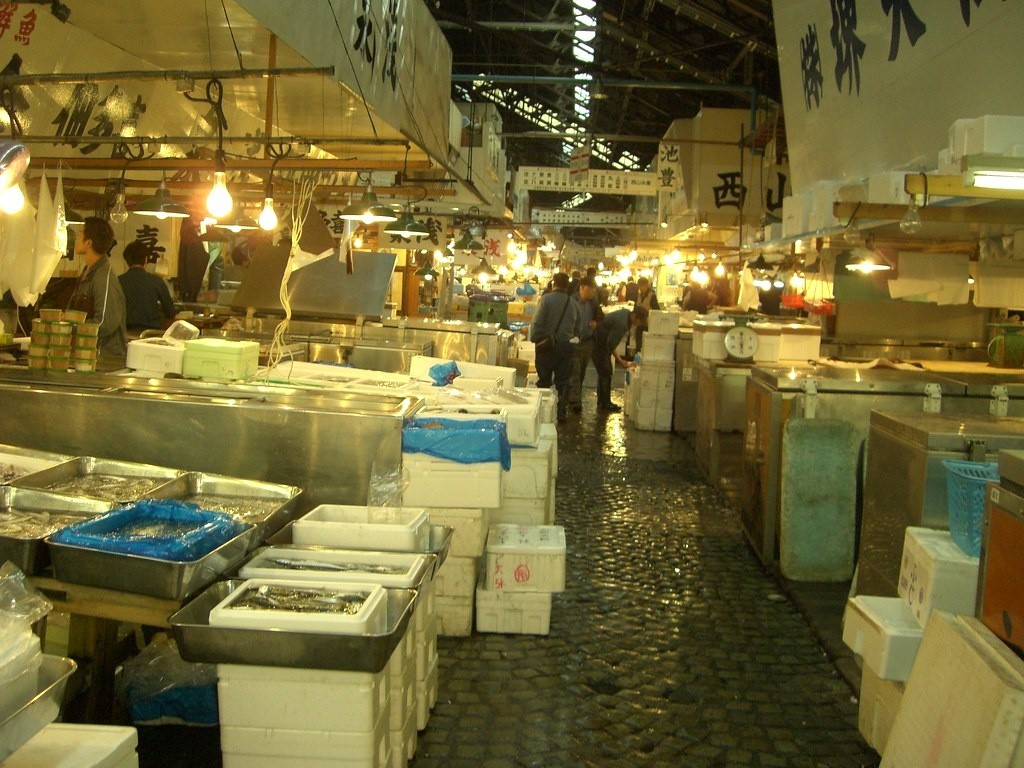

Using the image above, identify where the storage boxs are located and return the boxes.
[0,308,1024,768]
[764,112,1023,243]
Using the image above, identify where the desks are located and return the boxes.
[12,563,226,725]
[687,351,752,487]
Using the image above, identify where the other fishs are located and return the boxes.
[110,517,205,538]
[259,555,412,576]
[223,584,371,617]
[0,463,24,483]
[179,494,291,521]
[45,473,169,502]
[0,507,93,540]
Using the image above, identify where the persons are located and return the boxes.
[65,217,128,356]
[682,281,718,314]
[117,242,175,339]
[530,267,661,421]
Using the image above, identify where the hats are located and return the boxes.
[638,277,648,285]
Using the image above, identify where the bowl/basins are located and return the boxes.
[26,309,99,374]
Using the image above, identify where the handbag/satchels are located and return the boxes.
[535,335,555,361]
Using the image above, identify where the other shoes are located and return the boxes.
[597,401,621,413]
[558,415,566,422]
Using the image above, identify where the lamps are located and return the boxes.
[747,254,774,272]
[699,211,709,227]
[339,173,398,224]
[383,192,431,238]
[844,245,897,274]
[412,261,440,281]
[214,206,260,233]
[451,229,486,254]
[660,214,672,228]
[470,255,497,283]
[132,171,191,219]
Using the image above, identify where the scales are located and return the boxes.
[720,313,760,364]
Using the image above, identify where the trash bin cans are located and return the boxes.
[466,285,514,327]
